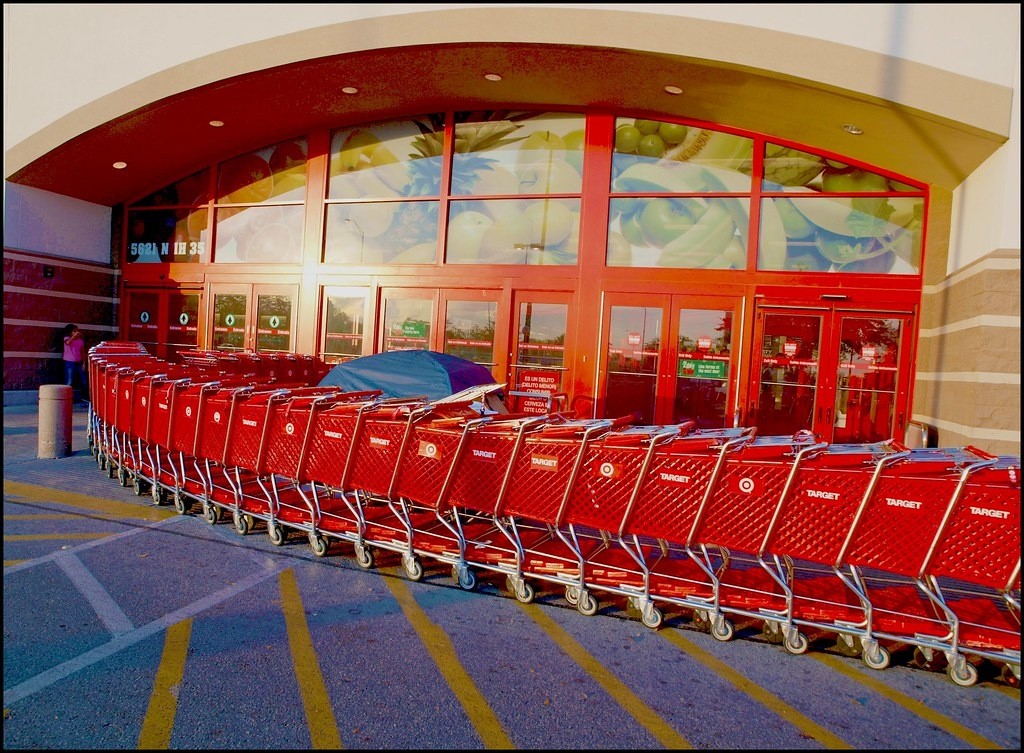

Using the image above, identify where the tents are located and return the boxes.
[318,349,505,415]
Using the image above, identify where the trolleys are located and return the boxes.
[84,339,1023,687]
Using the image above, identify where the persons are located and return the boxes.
[62,324,90,402]
[784,367,797,382]
[762,364,773,380]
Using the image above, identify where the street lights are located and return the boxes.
[346,217,364,267]
[516,242,546,265]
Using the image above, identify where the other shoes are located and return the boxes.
[82,396,90,402]
